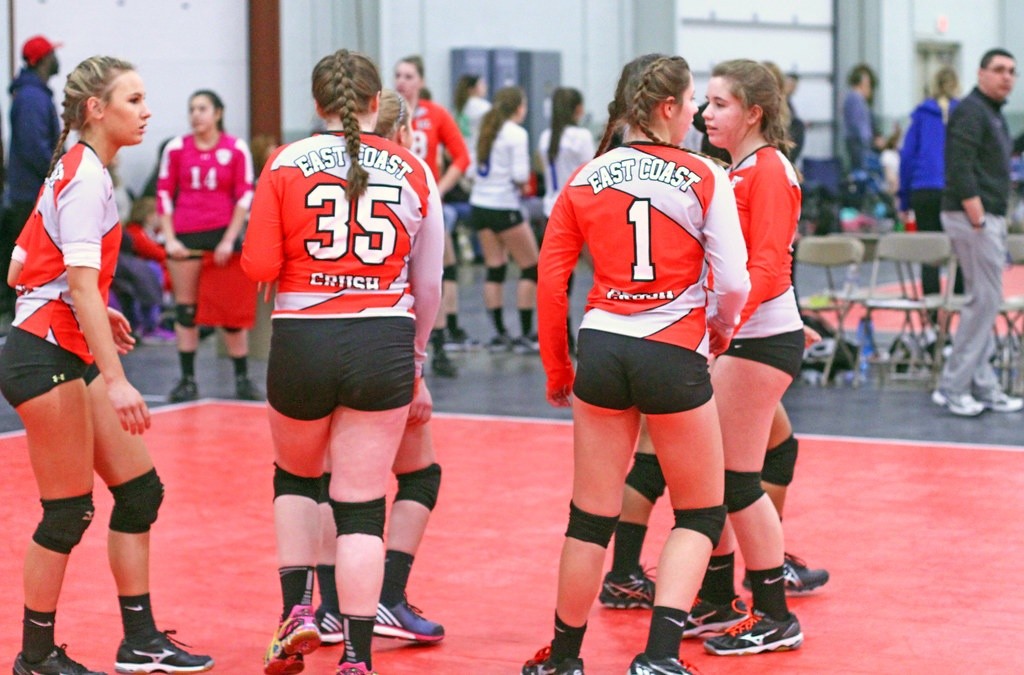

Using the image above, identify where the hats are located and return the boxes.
[24,35,62,64]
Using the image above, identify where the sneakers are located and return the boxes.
[114,628,214,672]
[743,553,829,596]
[373,600,443,640]
[705,609,802,656]
[12,643,105,675]
[682,589,748,638]
[317,611,345,643]
[599,562,656,609]
[263,604,321,675]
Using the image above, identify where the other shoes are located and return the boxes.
[236,381,266,402]
[974,390,1023,412]
[336,661,378,675]
[522,646,586,675]
[931,389,985,417]
[171,380,196,401]
[628,652,698,675]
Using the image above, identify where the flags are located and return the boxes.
[194,250,257,331]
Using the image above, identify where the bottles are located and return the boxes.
[857,316,875,370]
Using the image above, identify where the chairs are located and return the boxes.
[790,229,1024,400]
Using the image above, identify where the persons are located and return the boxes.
[1,36,1024,675]
